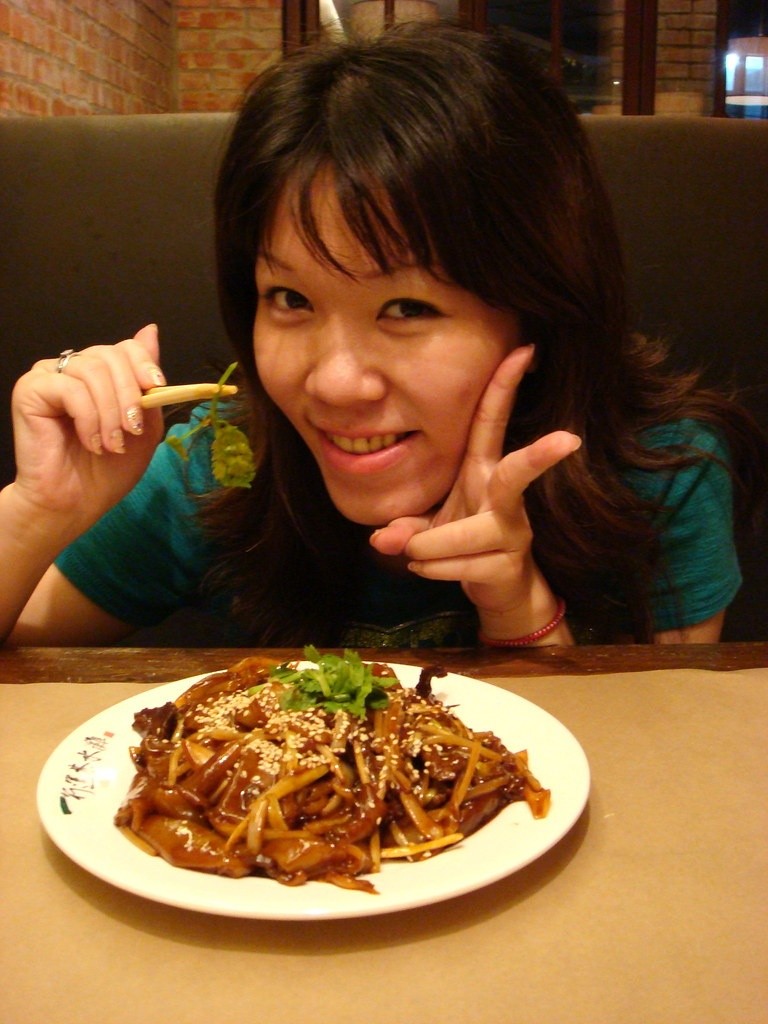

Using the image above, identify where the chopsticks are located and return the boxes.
[140,383,239,410]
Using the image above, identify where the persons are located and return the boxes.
[0,19,747,647]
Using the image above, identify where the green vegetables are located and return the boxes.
[267,646,401,718]
[165,361,257,487]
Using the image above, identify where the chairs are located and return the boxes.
[1,113,768,647]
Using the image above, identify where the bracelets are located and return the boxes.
[477,597,567,646]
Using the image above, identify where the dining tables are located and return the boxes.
[0,637,768,1024]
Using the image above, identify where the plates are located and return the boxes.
[37,661,591,920]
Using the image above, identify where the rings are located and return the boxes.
[57,349,82,373]
